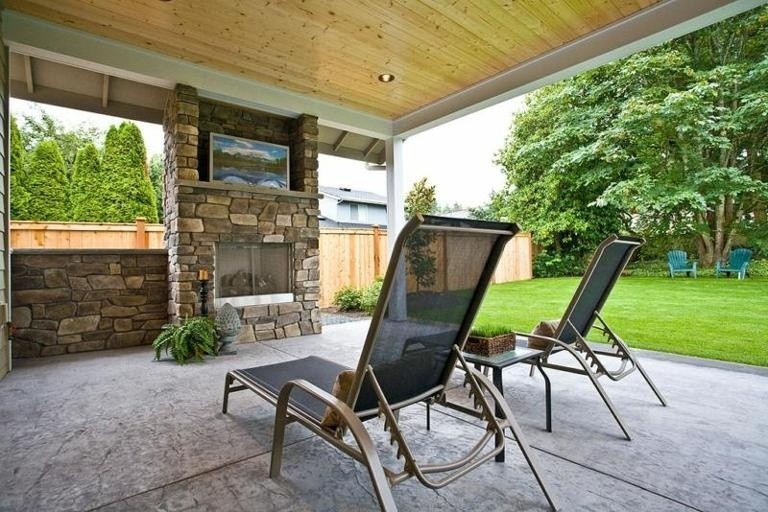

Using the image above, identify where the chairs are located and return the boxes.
[222,212,562,512]
[667,247,754,281]
[403,234,666,437]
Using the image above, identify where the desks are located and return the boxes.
[426,344,553,462]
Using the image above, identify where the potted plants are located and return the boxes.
[462,323,516,356]
[151,312,226,367]
